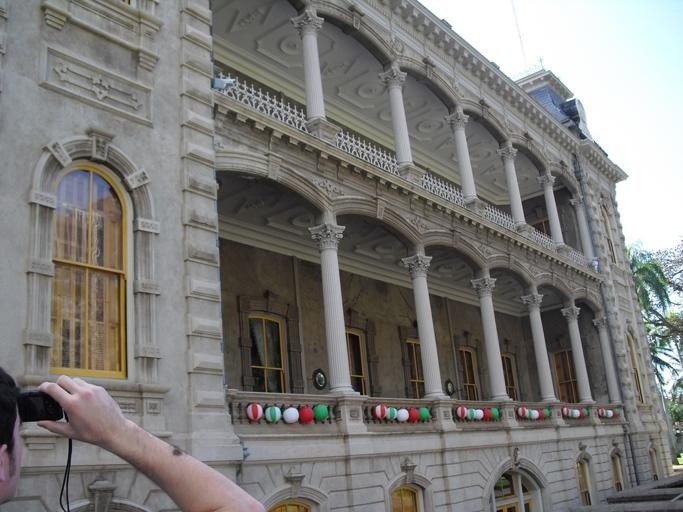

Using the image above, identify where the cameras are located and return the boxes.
[22,394,63,423]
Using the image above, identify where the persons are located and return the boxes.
[0,366,267,511]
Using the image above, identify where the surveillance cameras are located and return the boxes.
[591,256,601,261]
[212,77,236,92]
